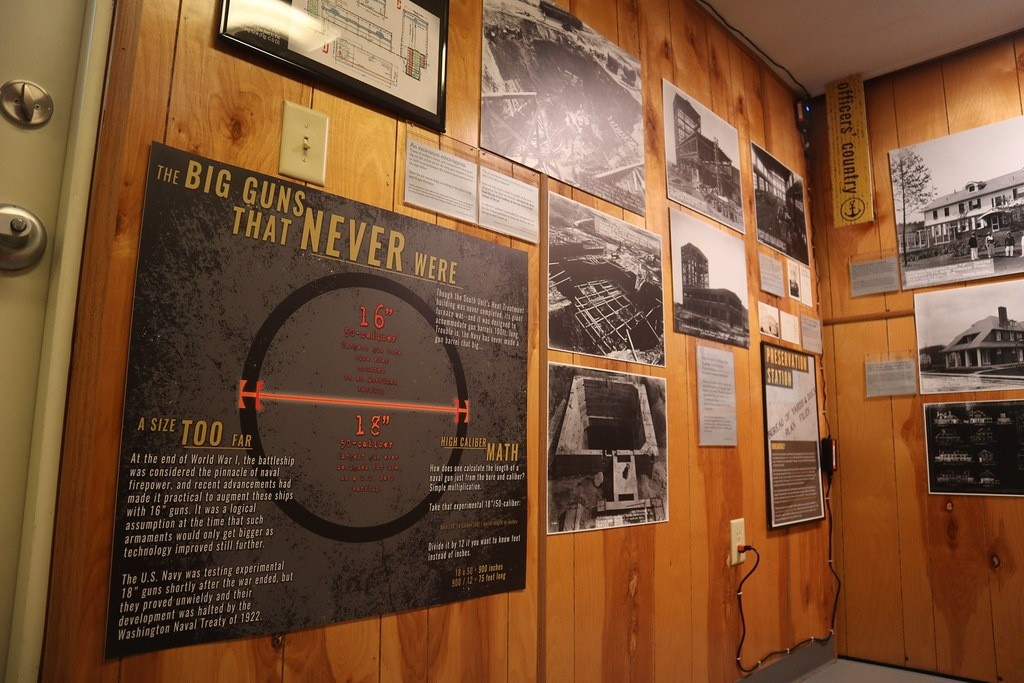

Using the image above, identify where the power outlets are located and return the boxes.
[729,518,747,565]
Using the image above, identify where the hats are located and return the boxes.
[987,231,991,234]
[971,231,975,235]
[1007,231,1011,235]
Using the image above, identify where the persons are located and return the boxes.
[1005,232,1016,257]
[1020,232,1024,258]
[985,232,995,258]
[969,232,980,260]
